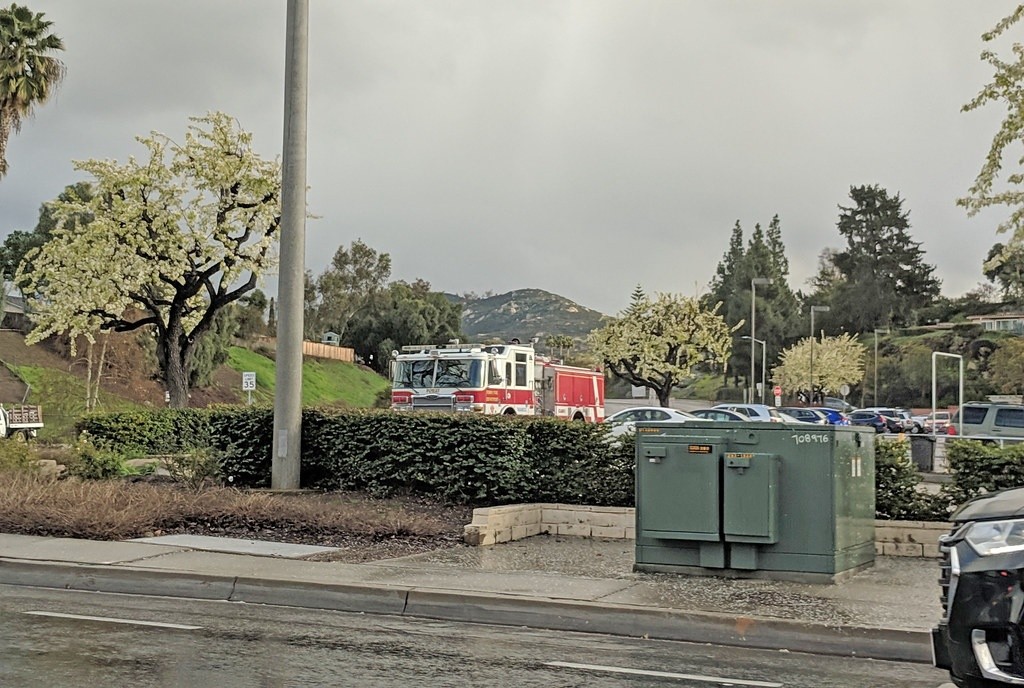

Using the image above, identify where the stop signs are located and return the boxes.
[773,386,782,396]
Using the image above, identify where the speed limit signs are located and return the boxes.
[242,371,257,392]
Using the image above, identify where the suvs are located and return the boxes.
[944,393,1024,452]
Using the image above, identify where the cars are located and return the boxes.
[927,485,1024,688]
[688,394,953,434]
[600,404,713,448]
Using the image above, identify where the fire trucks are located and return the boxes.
[387,337,606,425]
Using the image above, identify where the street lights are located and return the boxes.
[809,305,831,407]
[750,277,775,404]
[872,328,889,407]
[741,336,767,406]
